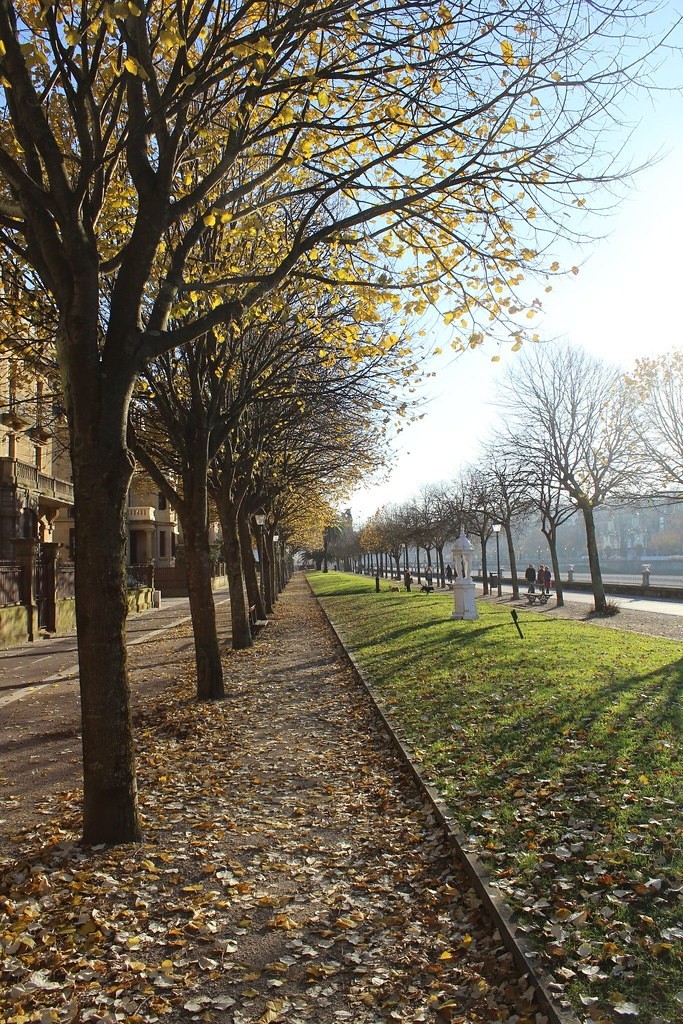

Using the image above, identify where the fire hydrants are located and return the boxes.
[375,575,380,593]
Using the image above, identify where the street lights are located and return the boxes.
[253,506,265,602]
[492,523,503,597]
[273,534,279,595]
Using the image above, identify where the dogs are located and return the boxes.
[389,586,400,592]
[420,586,434,595]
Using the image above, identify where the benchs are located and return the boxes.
[523,583,553,605]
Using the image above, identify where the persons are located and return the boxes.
[444,564,453,583]
[404,569,411,592]
[425,565,433,587]
[538,566,545,591]
[543,566,552,594]
[525,563,536,593]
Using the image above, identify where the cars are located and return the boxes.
[581,554,624,561]
